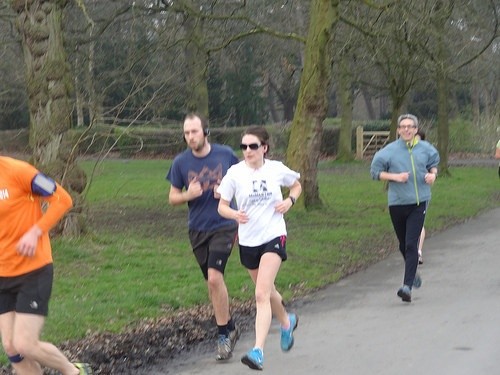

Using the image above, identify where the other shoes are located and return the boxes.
[418,251,423,265]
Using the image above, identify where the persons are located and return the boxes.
[166,112,241,363]
[414,130,428,263]
[495,127,500,177]
[216,125,303,371]
[0,157,92,375]
[371,114,440,302]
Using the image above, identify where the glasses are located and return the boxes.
[239,142,263,150]
[399,125,417,130]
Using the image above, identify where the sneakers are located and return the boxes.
[241,347,264,370]
[412,266,422,288]
[396,286,411,302]
[73,362,93,375]
[216,324,241,361]
[280,312,298,351]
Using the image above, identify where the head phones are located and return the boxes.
[183,128,210,142]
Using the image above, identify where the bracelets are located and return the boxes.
[290,196,296,206]
